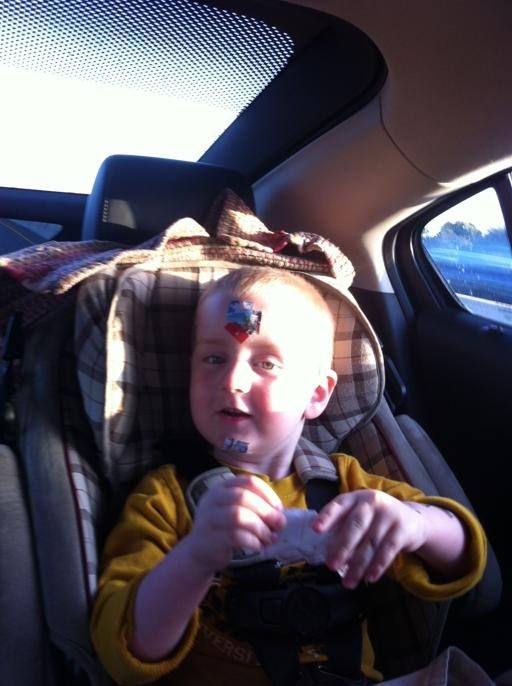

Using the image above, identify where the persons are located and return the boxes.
[83,264,493,684]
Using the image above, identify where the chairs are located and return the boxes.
[0,443,66,686]
[22,150,501,686]
